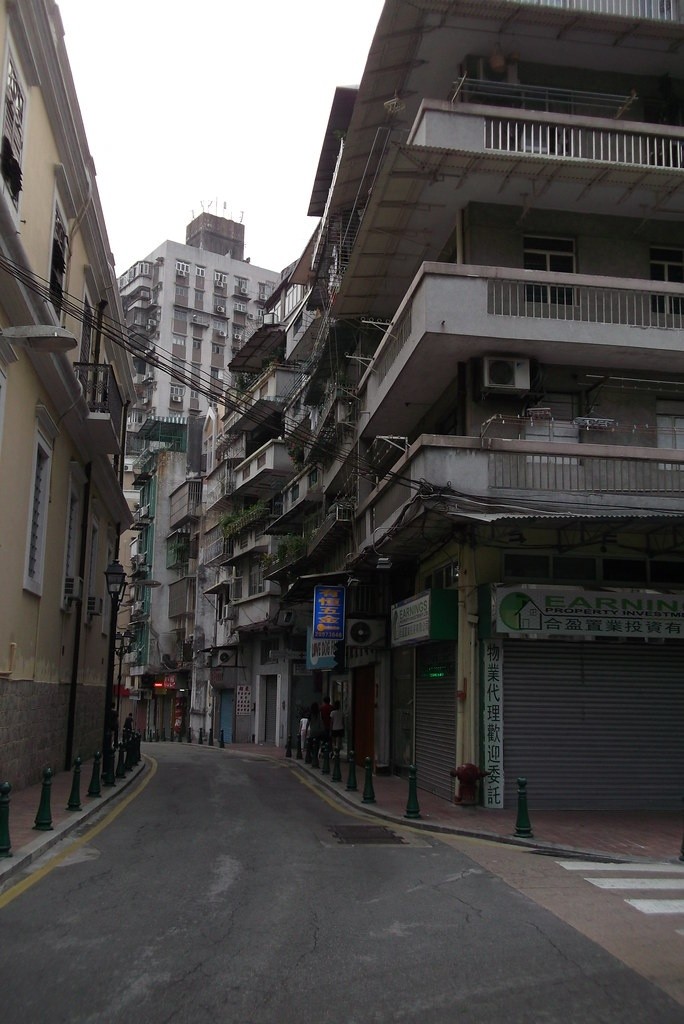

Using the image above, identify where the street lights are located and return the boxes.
[100,559,128,780]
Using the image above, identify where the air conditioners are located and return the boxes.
[241,287,247,294]
[217,281,224,288]
[124,465,133,472]
[141,290,149,298]
[173,396,181,402]
[222,604,238,620]
[211,649,236,668]
[478,353,531,401]
[63,575,84,603]
[219,330,226,337]
[141,372,154,383]
[146,319,157,332]
[259,293,268,301]
[216,305,225,313]
[88,595,104,617]
[247,313,254,320]
[139,506,148,518]
[292,662,314,677]
[234,333,241,340]
[141,398,148,404]
[345,618,388,650]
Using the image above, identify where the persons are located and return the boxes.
[111,701,134,751]
[300,696,344,764]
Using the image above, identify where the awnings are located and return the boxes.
[134,417,187,442]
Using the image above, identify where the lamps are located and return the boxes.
[347,577,362,589]
[147,377,153,385]
[376,557,392,569]
